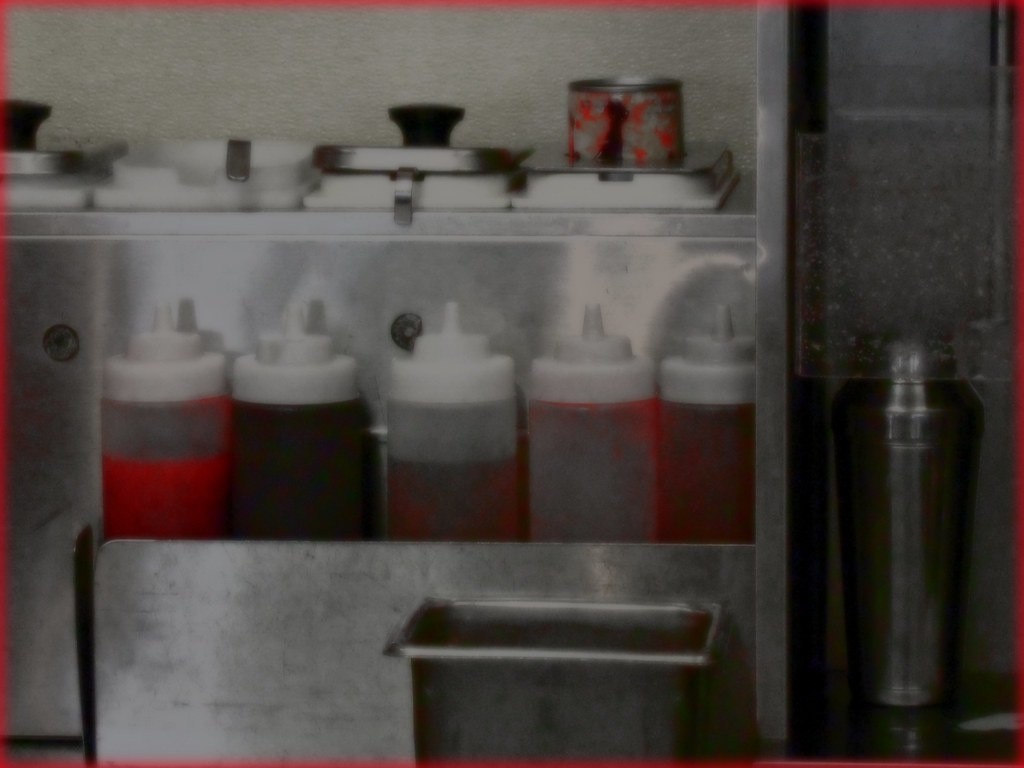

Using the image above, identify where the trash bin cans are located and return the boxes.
[832,337,983,711]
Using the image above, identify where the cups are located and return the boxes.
[568,75,682,169]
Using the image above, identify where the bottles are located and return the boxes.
[97,294,231,538]
[661,301,755,541]
[223,297,376,539]
[521,303,657,542]
[382,303,520,541]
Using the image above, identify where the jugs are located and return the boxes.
[832,338,985,712]
